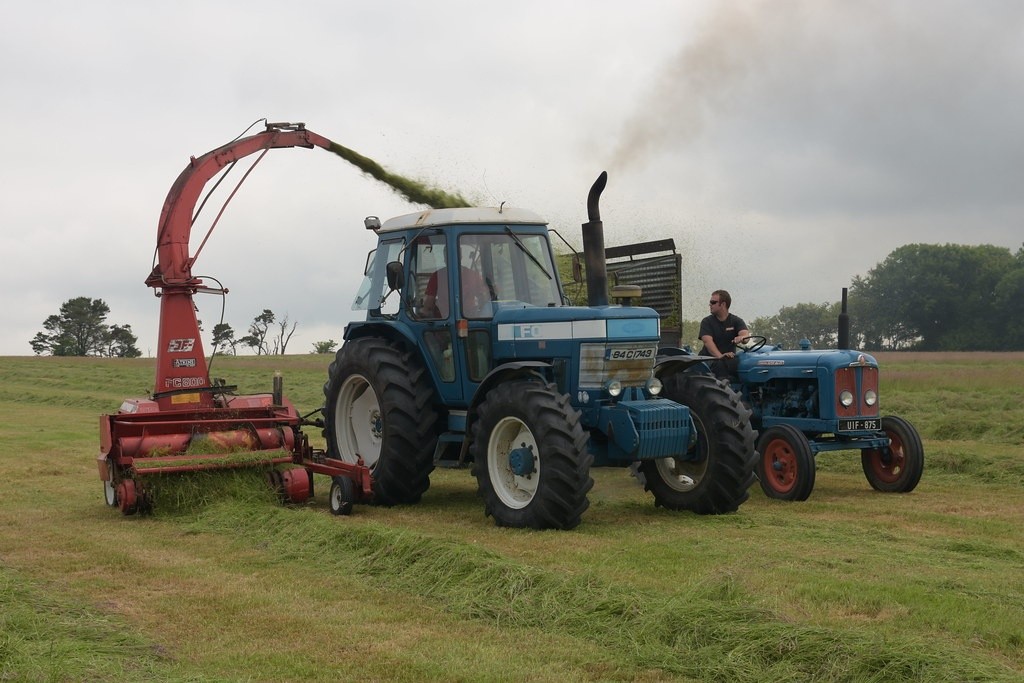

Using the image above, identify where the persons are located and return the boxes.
[698,290,750,358]
[424,243,489,380]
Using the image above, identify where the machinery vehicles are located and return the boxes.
[98,116,375,517]
[553,236,924,503]
[328,168,759,532]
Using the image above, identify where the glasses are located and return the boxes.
[710,300,721,305]
